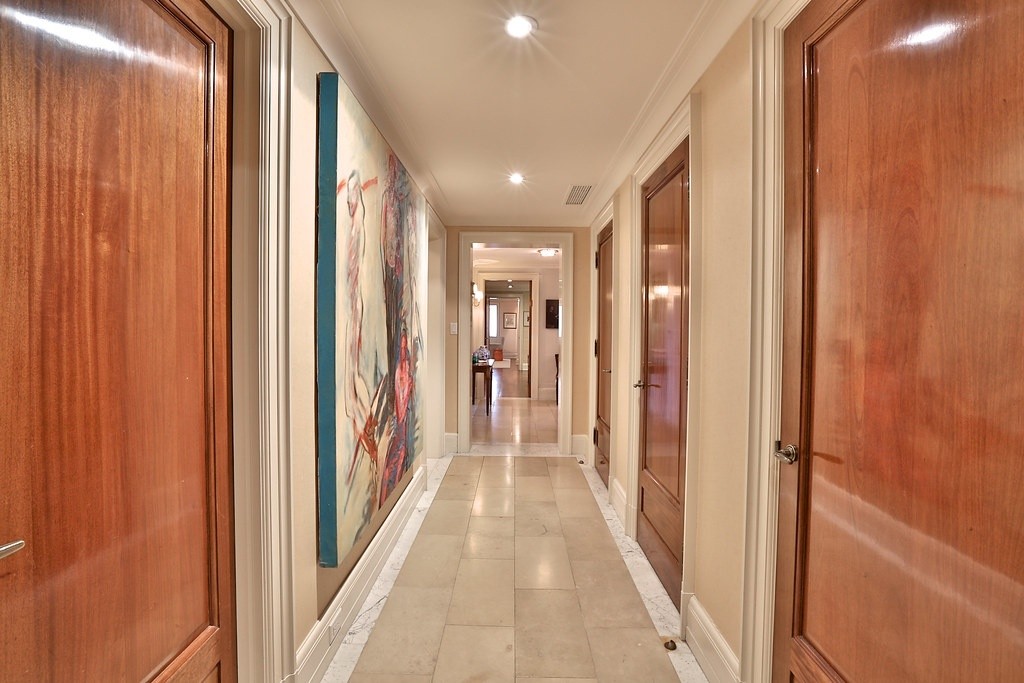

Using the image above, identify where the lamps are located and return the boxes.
[472,282,483,308]
[538,249,558,256]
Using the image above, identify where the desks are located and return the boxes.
[472,359,495,416]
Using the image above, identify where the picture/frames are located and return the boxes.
[523,311,529,327]
[503,313,517,329]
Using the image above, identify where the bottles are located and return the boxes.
[473,353,478,366]
[493,350,503,361]
